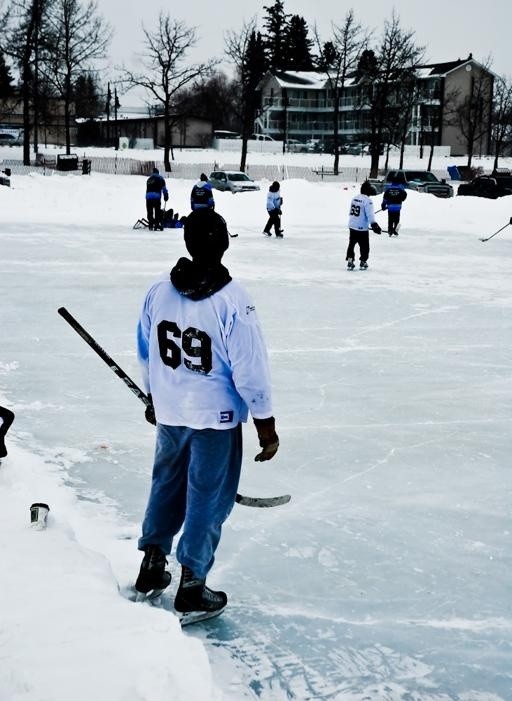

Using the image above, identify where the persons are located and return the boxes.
[263,180,284,237]
[134,208,279,615]
[145,168,169,231]
[343,181,381,269]
[190,173,215,210]
[376,177,407,237]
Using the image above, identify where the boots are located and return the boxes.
[174,566,228,612]
[135,544,172,593]
[360,260,368,266]
[347,257,355,268]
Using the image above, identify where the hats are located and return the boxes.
[184,208,229,259]
[200,172,208,181]
[361,180,371,195]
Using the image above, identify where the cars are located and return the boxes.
[338,143,400,155]
[208,171,260,194]
[307,138,325,153]
[215,130,274,141]
[284,139,309,152]
[0,133,24,147]
[458,174,511,199]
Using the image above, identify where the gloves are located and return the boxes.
[382,207,386,211]
[372,222,381,233]
[254,417,280,463]
[145,393,156,426]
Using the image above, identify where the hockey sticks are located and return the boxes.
[227,231,240,237]
[368,224,401,234]
[58,306,291,506]
[279,205,284,233]
[477,221,510,242]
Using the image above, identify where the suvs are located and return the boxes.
[383,169,453,197]
